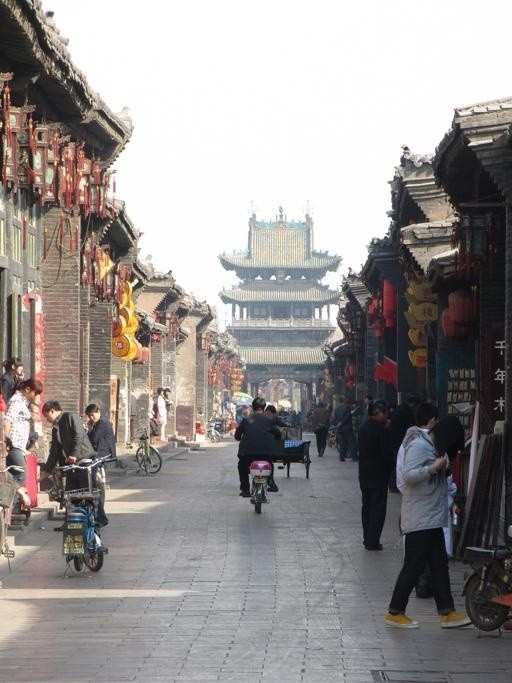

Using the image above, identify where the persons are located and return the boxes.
[150,387,174,443]
[383,413,474,630]
[234,392,417,552]
[395,402,457,598]
[84,402,117,528]
[0,357,43,518]
[37,400,99,531]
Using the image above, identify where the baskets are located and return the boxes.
[0,485,16,508]
[64,487,100,518]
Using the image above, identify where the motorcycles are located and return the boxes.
[54,454,118,577]
[462,523,512,638]
[240,460,272,514]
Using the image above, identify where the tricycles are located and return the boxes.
[275,422,311,479]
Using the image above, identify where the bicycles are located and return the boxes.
[208,416,220,444]
[0,464,25,570]
[135,427,162,474]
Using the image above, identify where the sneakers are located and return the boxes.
[267,479,279,491]
[239,490,250,496]
[365,545,383,551]
[440,612,472,628]
[54,526,64,531]
[385,612,419,628]
